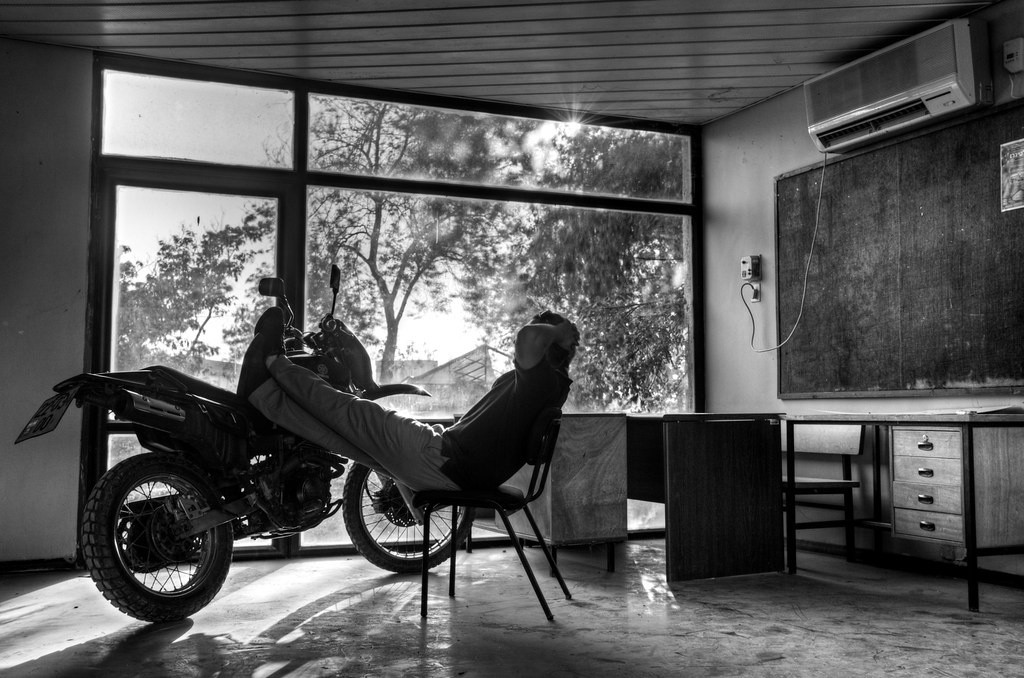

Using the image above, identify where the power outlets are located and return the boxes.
[751,283,761,303]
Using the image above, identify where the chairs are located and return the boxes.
[412,406,572,621]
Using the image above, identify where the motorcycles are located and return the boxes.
[15,263,479,623]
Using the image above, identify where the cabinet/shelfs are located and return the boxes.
[627,412,786,583]
[780,407,1024,612]
[452,412,628,578]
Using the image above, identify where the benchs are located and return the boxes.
[780,420,866,563]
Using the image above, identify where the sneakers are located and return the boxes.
[254,305,286,360]
[234,332,268,399]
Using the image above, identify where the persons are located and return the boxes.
[237,306,581,493]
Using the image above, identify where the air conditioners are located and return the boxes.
[803,18,995,155]
